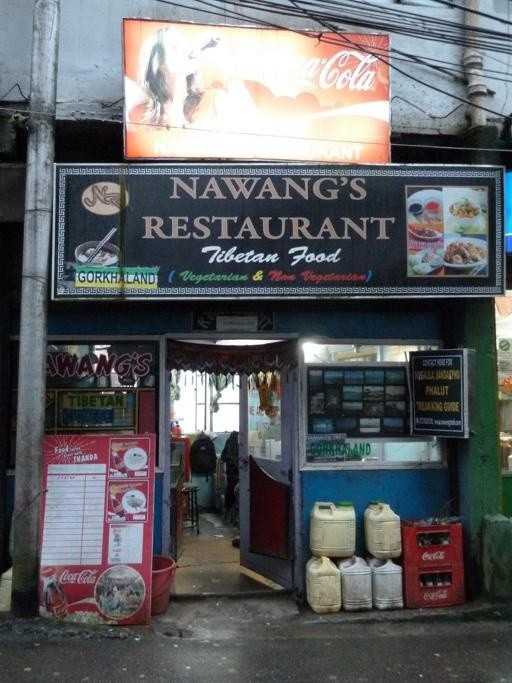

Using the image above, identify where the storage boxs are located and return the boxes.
[400,518,465,608]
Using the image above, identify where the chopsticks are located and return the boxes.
[85,226,118,263]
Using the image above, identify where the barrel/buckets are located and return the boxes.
[305,555,342,614]
[362,499,403,560]
[337,554,373,611]
[309,499,357,557]
[368,556,404,610]
[150,554,178,616]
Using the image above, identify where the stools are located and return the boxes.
[171,479,200,534]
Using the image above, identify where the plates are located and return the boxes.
[123,447,147,471]
[407,188,444,241]
[94,565,146,620]
[122,490,145,513]
[413,248,444,275]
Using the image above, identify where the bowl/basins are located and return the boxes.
[449,200,481,227]
[445,238,491,276]
[75,241,120,267]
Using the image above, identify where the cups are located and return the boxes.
[408,202,423,222]
[425,198,441,216]
